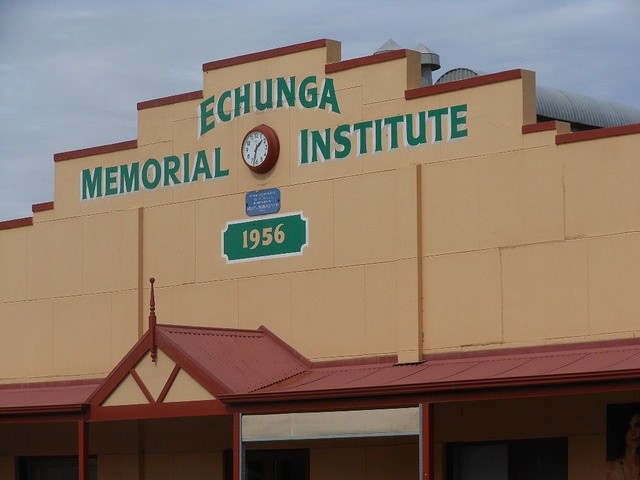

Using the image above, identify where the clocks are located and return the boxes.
[240,123,281,174]
